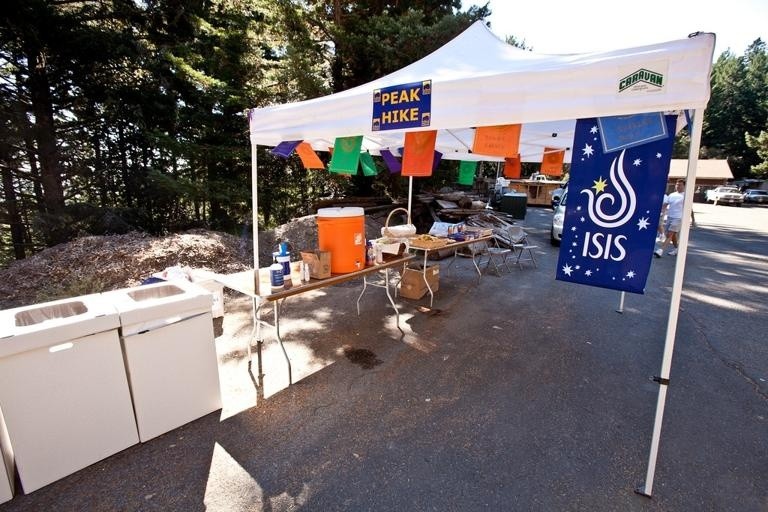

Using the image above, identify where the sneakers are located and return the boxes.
[659,233,665,240]
[655,249,663,257]
[668,248,678,256]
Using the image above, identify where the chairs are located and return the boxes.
[478,227,538,278]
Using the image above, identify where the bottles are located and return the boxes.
[448,224,462,234]
[376,245,383,263]
[270,259,284,289]
[365,241,373,266]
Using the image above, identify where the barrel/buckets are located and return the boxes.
[317,207,366,274]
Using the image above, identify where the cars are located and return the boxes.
[549,178,569,246]
[692,182,768,208]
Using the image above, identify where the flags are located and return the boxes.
[326,135,363,176]
[457,161,478,187]
[471,123,523,159]
[432,148,443,176]
[359,151,378,176]
[380,149,402,175]
[328,147,334,156]
[538,147,565,178]
[294,143,326,170]
[552,111,681,296]
[270,140,304,161]
[401,130,438,178]
[503,154,521,180]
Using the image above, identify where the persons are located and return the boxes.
[654,179,696,259]
[656,193,668,243]
